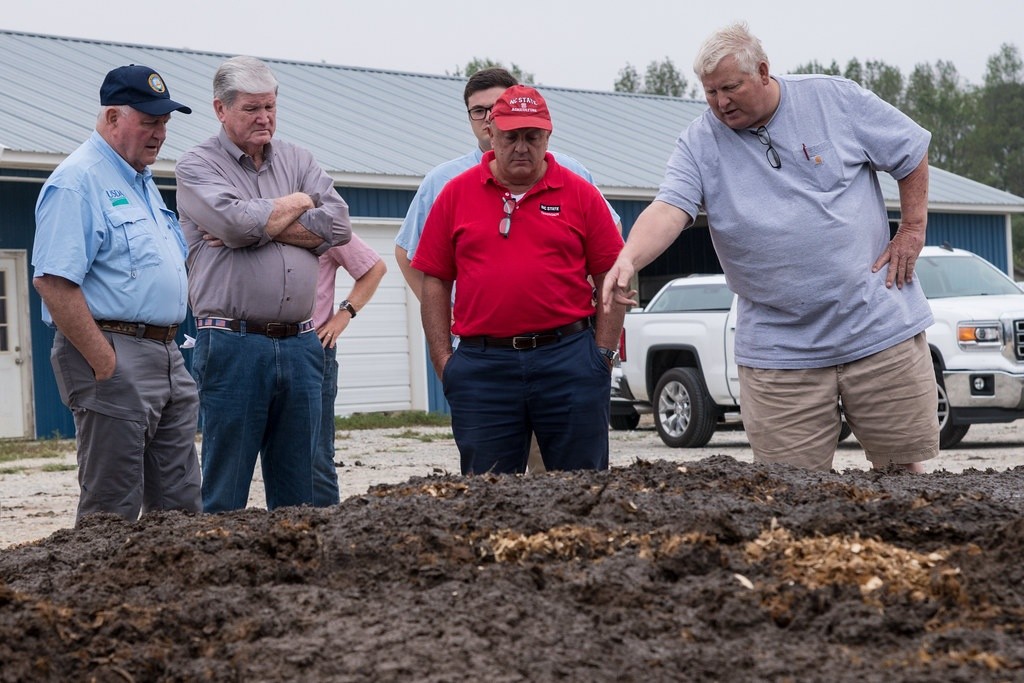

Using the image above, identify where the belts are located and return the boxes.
[197,316,315,338]
[460,316,592,350]
[95,320,178,343]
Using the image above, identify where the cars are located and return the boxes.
[608,272,736,432]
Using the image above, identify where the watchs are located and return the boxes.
[597,346,619,367]
[339,300,356,318]
[592,288,600,303]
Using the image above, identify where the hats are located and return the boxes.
[100,64,192,116]
[489,85,552,131]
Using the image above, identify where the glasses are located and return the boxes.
[468,106,493,121]
[499,197,516,238]
[750,125,782,169]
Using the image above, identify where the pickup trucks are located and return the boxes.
[618,243,1024,449]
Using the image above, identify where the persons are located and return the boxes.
[394,68,623,478]
[410,84,631,484]
[312,230,387,509]
[595,20,940,477]
[30,64,201,534]
[176,58,352,518]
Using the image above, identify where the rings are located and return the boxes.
[326,333,332,337]
